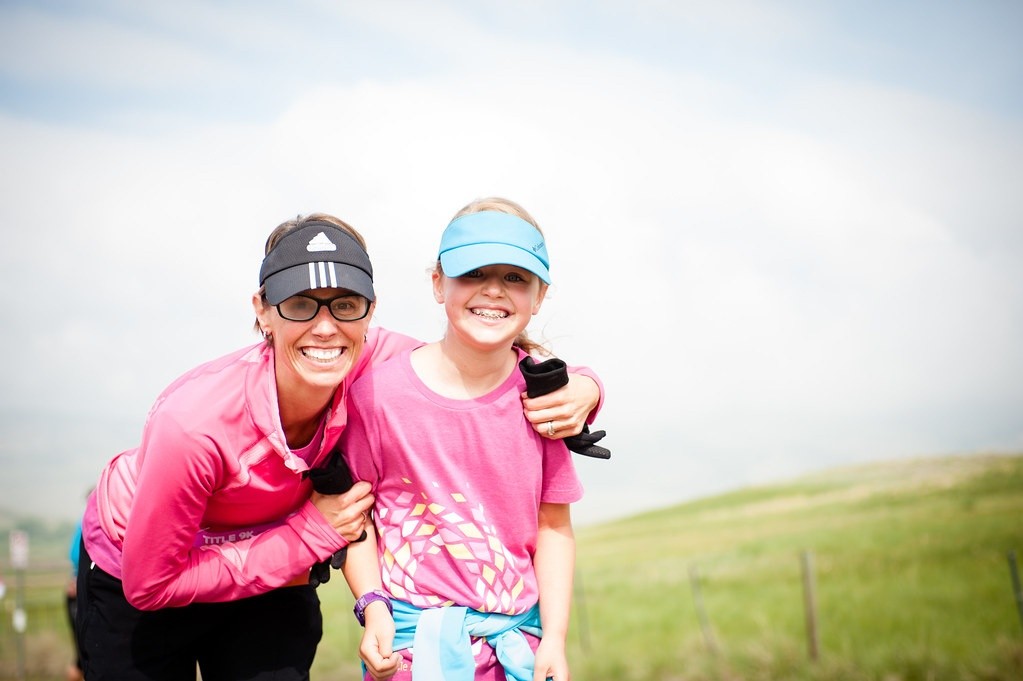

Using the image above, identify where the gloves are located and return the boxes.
[519,355,612,459]
[309,453,368,587]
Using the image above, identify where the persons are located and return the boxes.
[78,213,604,681]
[335,196,585,681]
[67,488,92,681]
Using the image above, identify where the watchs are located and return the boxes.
[352,589,393,626]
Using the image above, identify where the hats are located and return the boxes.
[437,210,551,284]
[259,221,373,306]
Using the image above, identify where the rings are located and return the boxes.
[362,512,367,523]
[547,422,555,436]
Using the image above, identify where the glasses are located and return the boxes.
[260,291,372,322]
